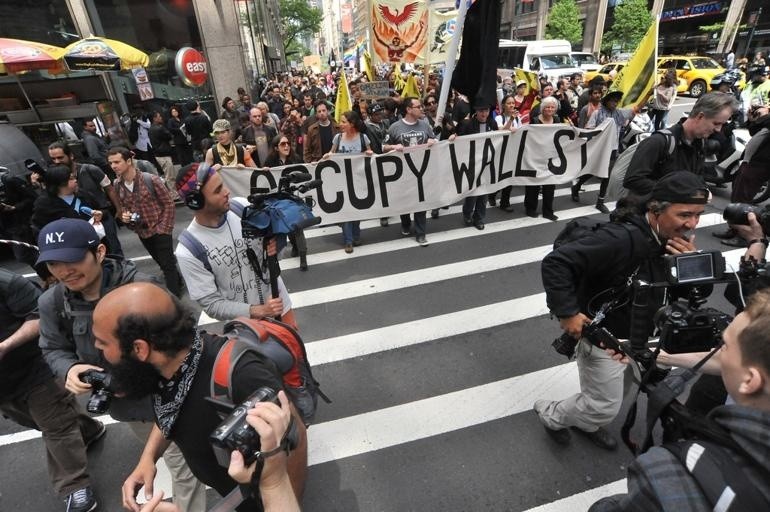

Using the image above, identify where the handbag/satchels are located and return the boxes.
[128,122,138,145]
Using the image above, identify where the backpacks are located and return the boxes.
[551,216,642,254]
[606,128,676,202]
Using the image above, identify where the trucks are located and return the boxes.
[496,40,584,92]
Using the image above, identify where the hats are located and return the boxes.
[516,80,527,88]
[642,169,708,204]
[222,96,232,109]
[588,74,613,90]
[600,88,624,107]
[367,102,384,116]
[213,119,231,134]
[35,216,102,266]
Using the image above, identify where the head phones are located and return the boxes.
[184,163,209,210]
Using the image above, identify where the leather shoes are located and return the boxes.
[712,228,747,247]
[587,426,618,448]
[534,400,571,443]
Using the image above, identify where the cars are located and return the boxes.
[433,68,445,77]
[571,51,747,98]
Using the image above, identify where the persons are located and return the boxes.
[533,46,769,512]
[0,97,307,512]
[129,61,535,272]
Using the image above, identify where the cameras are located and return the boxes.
[127,212,140,225]
[78,371,121,414]
[723,201,769,239]
[203,386,284,471]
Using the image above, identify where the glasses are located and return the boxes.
[278,141,291,147]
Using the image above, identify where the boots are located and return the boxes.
[596,197,610,214]
[571,183,581,202]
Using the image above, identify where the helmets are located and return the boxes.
[744,62,769,84]
[710,69,741,92]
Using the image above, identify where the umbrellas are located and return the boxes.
[48,34,150,101]
[0,36,71,111]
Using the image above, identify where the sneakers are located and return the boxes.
[431,210,439,216]
[462,211,472,223]
[401,224,411,234]
[84,416,106,451]
[380,217,388,227]
[300,251,309,271]
[353,236,360,245]
[543,211,558,221]
[416,234,428,246]
[500,202,513,212]
[173,197,184,204]
[291,245,298,257]
[63,485,98,512]
[525,206,539,217]
[488,195,496,206]
[212,315,319,428]
[345,243,353,253]
[474,212,484,230]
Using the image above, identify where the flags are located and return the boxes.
[450,0,504,114]
[362,51,373,81]
[604,20,657,113]
